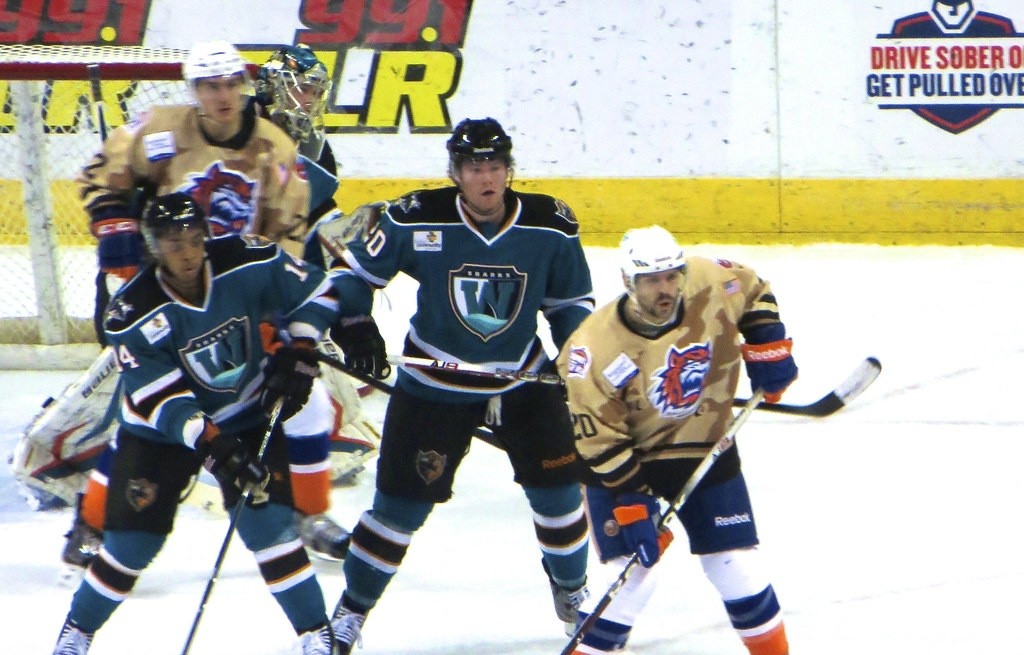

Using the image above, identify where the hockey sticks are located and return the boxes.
[87,60,112,146]
[180,396,286,654]
[559,386,767,655]
[384,352,884,421]
[353,417,382,450]
[317,347,504,451]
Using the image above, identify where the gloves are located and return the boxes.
[91,209,146,283]
[612,492,674,569]
[329,311,390,380]
[194,432,275,509]
[258,349,321,422]
[739,339,799,406]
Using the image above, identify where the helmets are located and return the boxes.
[141,192,205,228]
[619,225,685,304]
[182,41,246,79]
[258,46,330,144]
[447,116,513,162]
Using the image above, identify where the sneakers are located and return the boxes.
[330,597,367,655]
[541,558,596,639]
[52,612,93,655]
[61,519,104,583]
[297,510,349,563]
[300,621,335,655]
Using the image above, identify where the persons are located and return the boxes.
[260,115,595,655]
[571,224,800,655]
[52,192,341,655]
[8,36,383,512]
[255,45,341,269]
